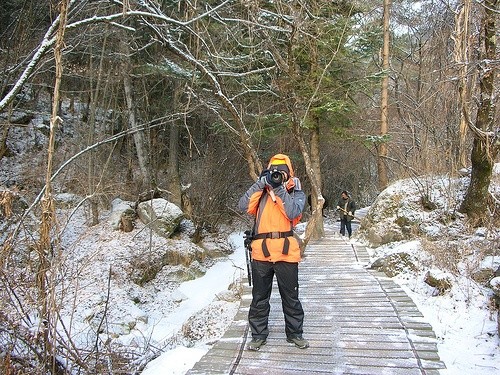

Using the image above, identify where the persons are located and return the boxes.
[337,191,356,239]
[308,188,323,216]
[238,153,309,351]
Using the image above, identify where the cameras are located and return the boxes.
[268,168,287,184]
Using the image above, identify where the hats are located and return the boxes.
[267,154,293,177]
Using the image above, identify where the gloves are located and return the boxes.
[336,206,340,209]
[260,169,269,182]
[348,211,352,216]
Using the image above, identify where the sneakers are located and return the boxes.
[286,336,309,348]
[247,339,267,350]
